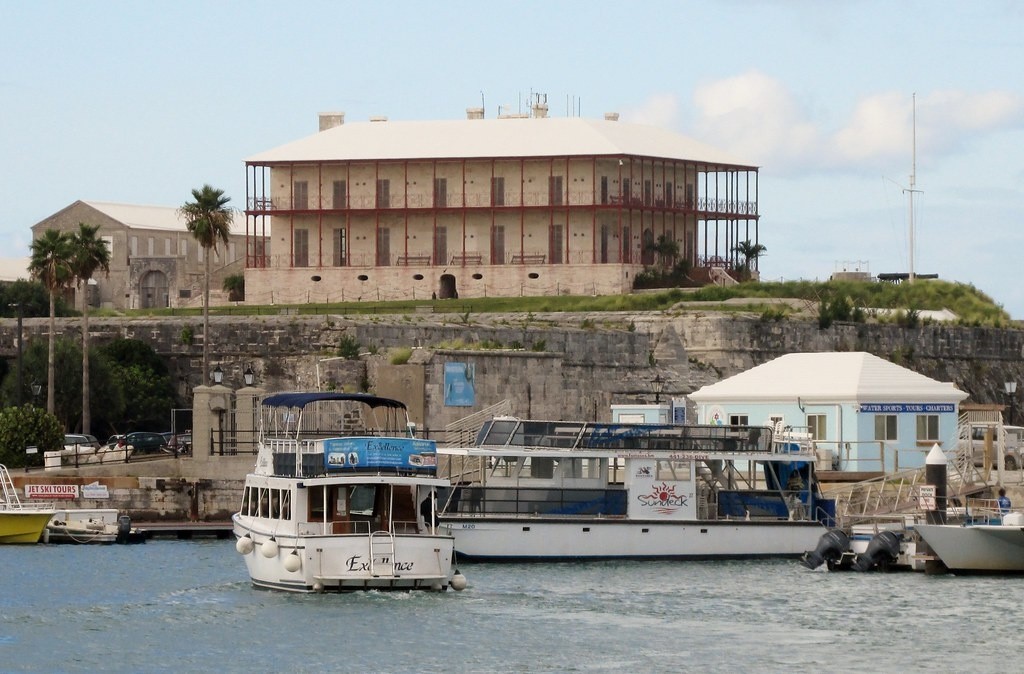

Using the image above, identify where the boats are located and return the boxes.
[232,392,468,594]
[436,414,836,556]
[0,465,147,545]
[913,499,1024,572]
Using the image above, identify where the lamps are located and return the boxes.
[213,362,224,386]
[244,364,255,387]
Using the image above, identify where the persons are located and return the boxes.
[419,491,440,535]
[994,489,1012,517]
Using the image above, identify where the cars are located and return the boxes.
[63,428,193,464]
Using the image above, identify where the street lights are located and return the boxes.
[1004,378,1017,425]
[650,374,665,403]
[31,378,43,408]
